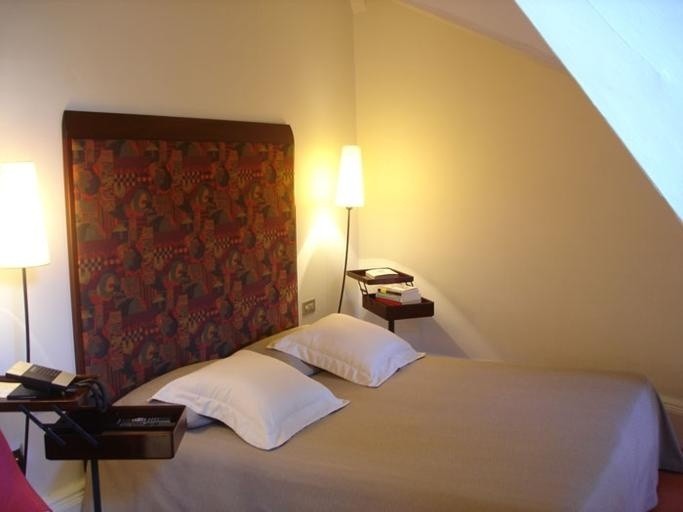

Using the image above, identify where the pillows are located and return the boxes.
[147,349,351,452]
[243,325,324,376]
[114,359,229,429]
[266,312,426,389]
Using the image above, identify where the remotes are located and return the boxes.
[116,416,172,428]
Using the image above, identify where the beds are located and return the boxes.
[61,109,683,512]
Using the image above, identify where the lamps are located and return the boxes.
[333,145,364,313]
[0,159,51,364]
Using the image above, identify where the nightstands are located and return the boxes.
[348,267,434,332]
[0,375,100,512]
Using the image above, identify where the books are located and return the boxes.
[376,284,422,306]
[0,382,41,399]
[366,269,399,279]
